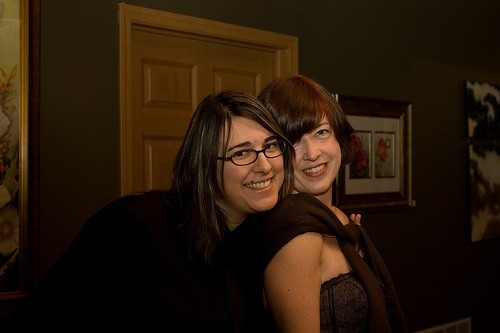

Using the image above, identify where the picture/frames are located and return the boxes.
[330,92,417,217]
[0,0,43,306]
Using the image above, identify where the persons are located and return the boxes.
[18,90,360,332]
[256,74,400,332]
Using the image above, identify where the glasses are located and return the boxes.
[217,139,287,166]
